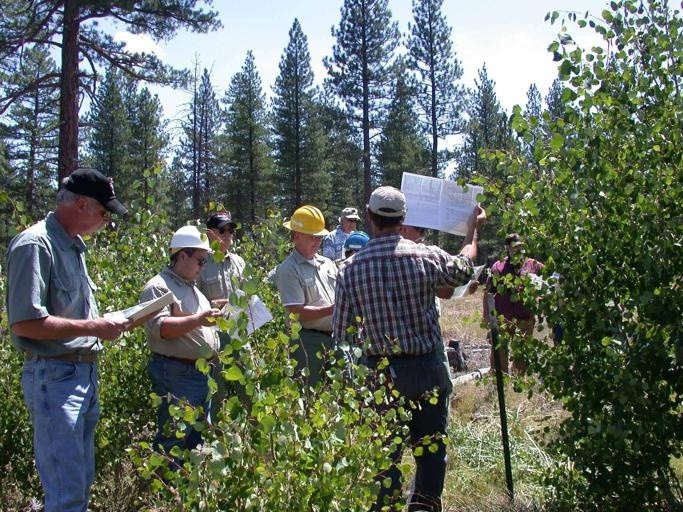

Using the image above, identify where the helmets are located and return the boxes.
[344,230,370,251]
[167,224,212,253]
[282,204,331,236]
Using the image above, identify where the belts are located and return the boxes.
[302,325,336,336]
[24,350,101,365]
[150,351,219,364]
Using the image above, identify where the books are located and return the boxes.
[101,291,174,327]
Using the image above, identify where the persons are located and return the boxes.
[6,169,163,510]
[482,233,547,379]
[331,184,485,512]
[140,225,226,500]
[401,223,480,511]
[196,210,264,443]
[546,265,570,351]
[273,205,370,389]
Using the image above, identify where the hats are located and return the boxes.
[340,207,361,219]
[365,185,408,218]
[504,233,525,248]
[60,165,128,217]
[205,211,241,230]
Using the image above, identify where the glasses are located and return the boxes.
[190,253,208,267]
[218,226,236,235]
